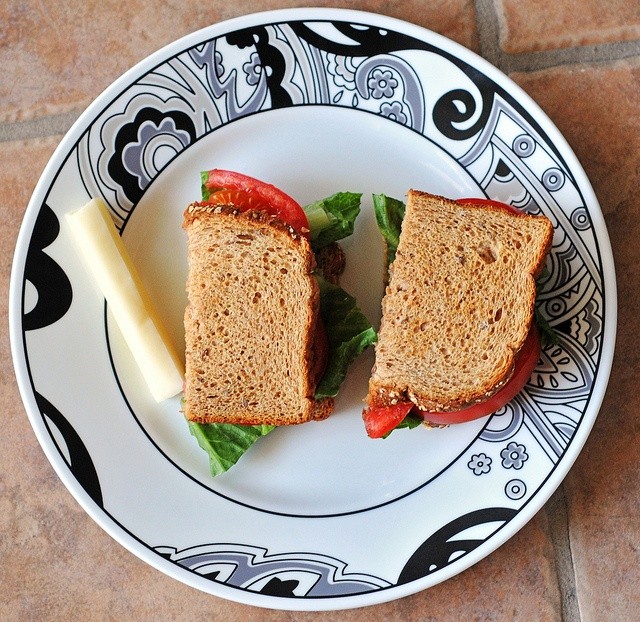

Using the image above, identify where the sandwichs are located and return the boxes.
[181,170,378,478]
[365,191,555,439]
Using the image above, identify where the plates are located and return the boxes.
[7,6,620,613]
